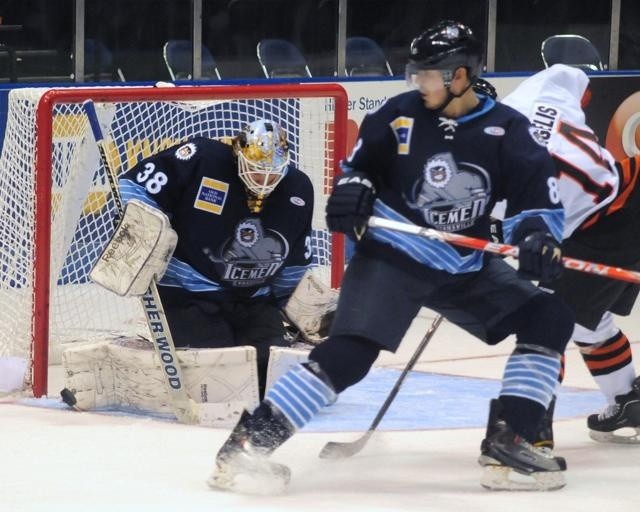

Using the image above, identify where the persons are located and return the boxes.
[204,19,568,485]
[55,118,314,428]
[472,62,640,446]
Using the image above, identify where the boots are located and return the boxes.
[216,403,292,486]
[480,398,568,475]
[532,397,554,449]
[587,377,640,431]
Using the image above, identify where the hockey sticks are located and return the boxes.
[317,312,444,459]
[82,98,255,428]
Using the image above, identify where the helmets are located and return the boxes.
[404,19,487,89]
[233,119,291,198]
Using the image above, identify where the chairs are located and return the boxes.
[0,0,606,82]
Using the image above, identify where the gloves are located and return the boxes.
[326,169,382,241]
[513,216,566,288]
